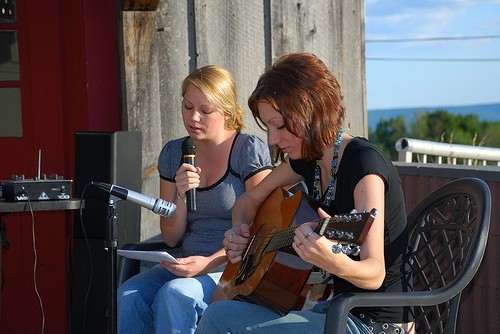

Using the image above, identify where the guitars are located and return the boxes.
[210,186,378,315]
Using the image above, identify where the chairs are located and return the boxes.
[323,177,491,334]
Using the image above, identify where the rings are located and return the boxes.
[305,231,313,239]
[296,243,303,247]
[227,232,235,242]
[225,248,230,256]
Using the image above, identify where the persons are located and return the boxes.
[117,66,273,334]
[194,53,415,334]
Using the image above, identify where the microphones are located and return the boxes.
[182,138,196,213]
[91,181,177,217]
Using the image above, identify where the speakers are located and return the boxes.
[66,129,141,334]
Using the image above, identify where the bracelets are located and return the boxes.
[177,191,187,202]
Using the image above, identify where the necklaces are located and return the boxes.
[313,128,343,208]
